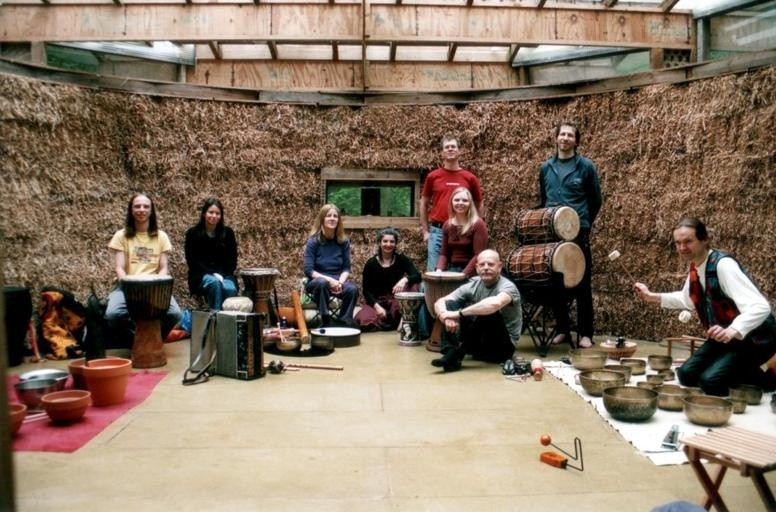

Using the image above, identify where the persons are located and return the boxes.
[106,193,183,345]
[303,203,359,327]
[353,229,423,333]
[430,248,523,372]
[183,196,239,307]
[539,122,603,348]
[418,135,484,270]
[435,187,490,279]
[632,216,776,397]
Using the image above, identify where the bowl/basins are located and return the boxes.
[312,338,335,355]
[7,368,90,436]
[569,345,764,425]
[275,335,302,353]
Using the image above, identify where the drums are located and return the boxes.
[122,275,174,368]
[393,292,425,347]
[515,206,580,242]
[423,271,465,352]
[311,326,362,348]
[241,268,280,327]
[507,242,586,288]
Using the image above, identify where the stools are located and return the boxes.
[306,293,343,329]
[679,427,776,511]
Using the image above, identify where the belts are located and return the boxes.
[431,222,443,228]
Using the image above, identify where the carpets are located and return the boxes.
[542,356,776,466]
[6,370,169,453]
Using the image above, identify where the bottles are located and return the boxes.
[281,315,286,328]
[531,357,544,382]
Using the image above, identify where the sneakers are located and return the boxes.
[502,360,514,375]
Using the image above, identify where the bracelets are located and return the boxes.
[458,308,464,317]
